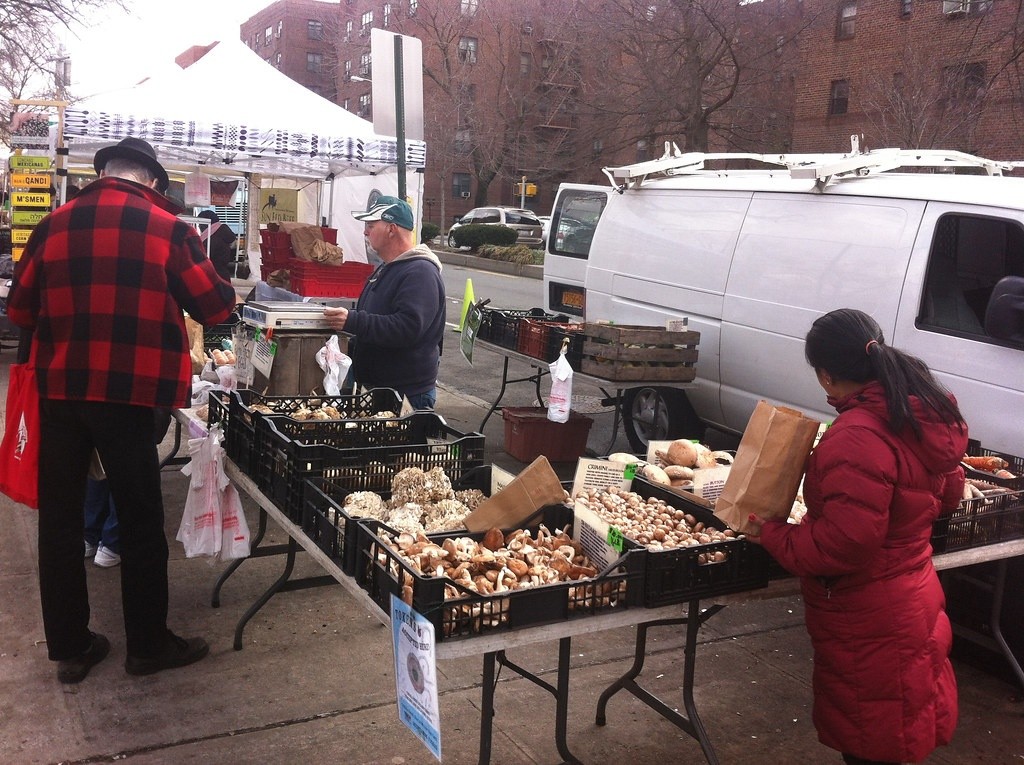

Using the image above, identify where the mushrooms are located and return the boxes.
[369,439,806,638]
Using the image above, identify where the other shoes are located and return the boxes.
[93,541,121,567]
[84,541,98,557]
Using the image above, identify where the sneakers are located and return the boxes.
[125,628,209,676]
[57,631,110,684]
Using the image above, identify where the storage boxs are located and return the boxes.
[237,324,356,397]
[208,387,1024,641]
[257,227,375,299]
[476,307,701,383]
[201,312,242,359]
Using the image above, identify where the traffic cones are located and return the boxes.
[453,279,476,332]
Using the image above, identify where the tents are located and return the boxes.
[53,34,426,278]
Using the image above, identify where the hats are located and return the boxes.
[94,137,169,192]
[351,196,414,231]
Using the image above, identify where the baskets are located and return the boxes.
[289,256,375,298]
[924,443,1024,556]
[207,379,801,643]
[476,306,614,371]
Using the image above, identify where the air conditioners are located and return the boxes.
[360,68,368,75]
[461,192,470,197]
[524,27,531,33]
[358,30,365,36]
[468,57,476,63]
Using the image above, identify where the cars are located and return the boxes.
[447,206,544,249]
[538,216,590,244]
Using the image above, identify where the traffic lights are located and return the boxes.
[513,184,522,195]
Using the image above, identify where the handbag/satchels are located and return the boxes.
[0,329,41,509]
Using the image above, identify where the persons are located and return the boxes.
[5,137,239,684]
[745,308,969,765]
[324,196,446,412]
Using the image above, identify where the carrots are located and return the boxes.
[951,455,1023,544]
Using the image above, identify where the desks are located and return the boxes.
[474,336,701,461]
[157,395,1023,765]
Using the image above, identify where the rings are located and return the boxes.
[331,321,333,325]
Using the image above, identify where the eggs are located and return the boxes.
[212,348,235,364]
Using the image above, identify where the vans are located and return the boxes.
[542,133,1024,474]
